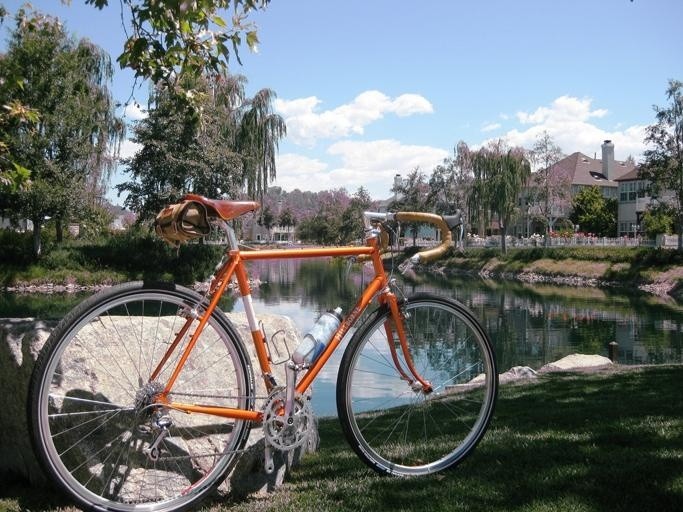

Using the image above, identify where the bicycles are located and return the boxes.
[27,192,498,511]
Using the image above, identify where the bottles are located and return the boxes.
[292,306,344,367]
